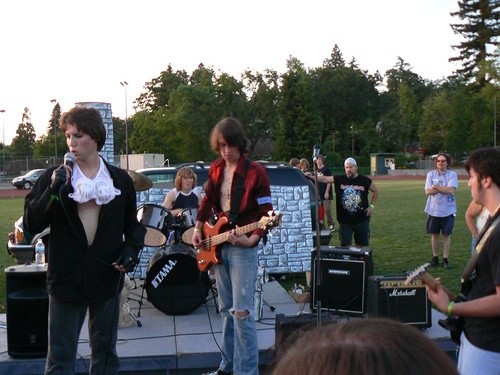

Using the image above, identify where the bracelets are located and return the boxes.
[370,204,375,208]
[193,228,202,232]
[447,300,454,317]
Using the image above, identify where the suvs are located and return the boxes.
[405,142,440,160]
[137,161,333,248]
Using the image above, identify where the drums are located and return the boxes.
[145,244,209,315]
[136,205,173,247]
[179,209,213,245]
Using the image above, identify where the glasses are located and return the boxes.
[436,160,446,163]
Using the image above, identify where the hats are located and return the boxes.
[345,157,356,165]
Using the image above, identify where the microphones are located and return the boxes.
[313,143,321,159]
[64,151,76,173]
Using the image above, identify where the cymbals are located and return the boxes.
[130,171,151,193]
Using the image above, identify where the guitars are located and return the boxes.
[197,210,284,272]
[404,263,463,346]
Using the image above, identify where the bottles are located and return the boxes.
[35,239,45,266]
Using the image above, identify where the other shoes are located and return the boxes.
[202,368,233,375]
[329,224,334,232]
[443,261,451,269]
[428,260,439,266]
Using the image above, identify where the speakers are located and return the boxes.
[367,273,433,329]
[310,245,371,315]
[8,289,51,357]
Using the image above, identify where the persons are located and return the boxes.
[190,117,275,375]
[304,157,379,246]
[417,147,500,375]
[463,199,490,259]
[289,156,336,232]
[158,166,208,306]
[262,315,460,375]
[423,153,458,270]
[22,107,147,375]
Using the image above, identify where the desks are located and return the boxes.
[6,239,36,266]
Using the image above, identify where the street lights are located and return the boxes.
[50,99,56,163]
[0,109,6,170]
[122,82,129,174]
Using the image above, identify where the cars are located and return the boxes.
[0,171,8,176]
[12,169,48,190]
[431,151,450,160]
